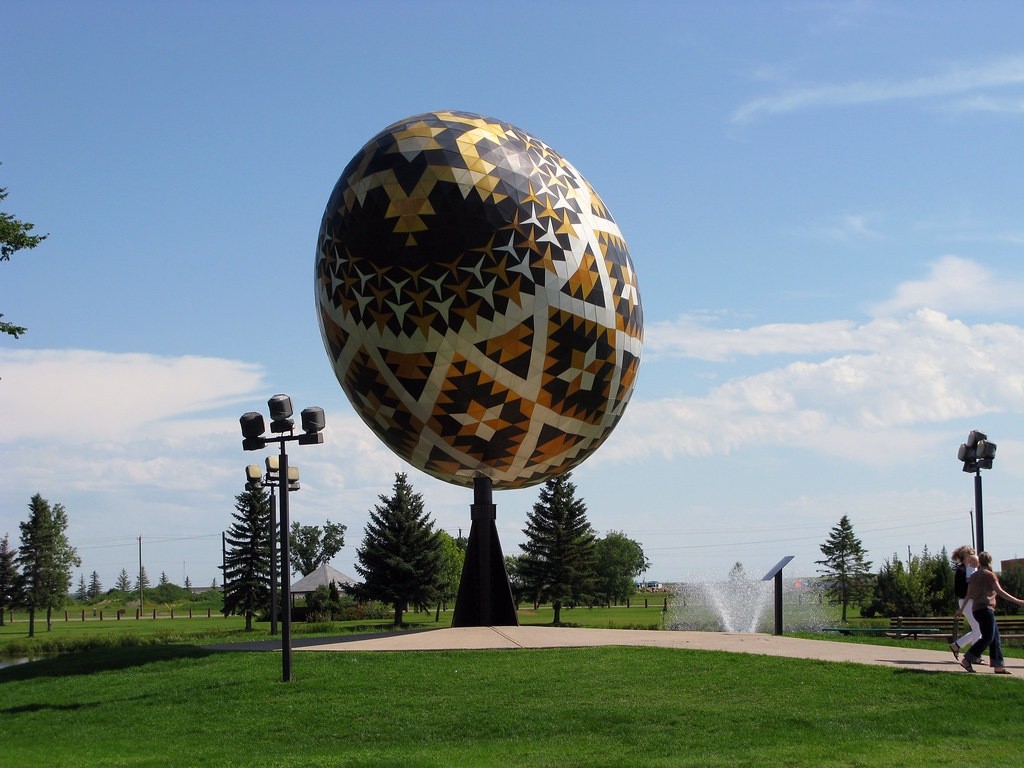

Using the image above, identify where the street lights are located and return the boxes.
[957,429,997,555]
[245,457,300,635]
[239,393,326,681]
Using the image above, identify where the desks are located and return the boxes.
[822,628,939,640]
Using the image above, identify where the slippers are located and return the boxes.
[950,645,959,661]
[960,662,976,672]
[995,668,1012,674]
[972,659,987,665]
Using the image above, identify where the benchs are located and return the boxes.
[995,620,1024,638]
[885,617,964,643]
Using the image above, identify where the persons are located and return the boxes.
[954,552,1024,674]
[949,547,989,666]
